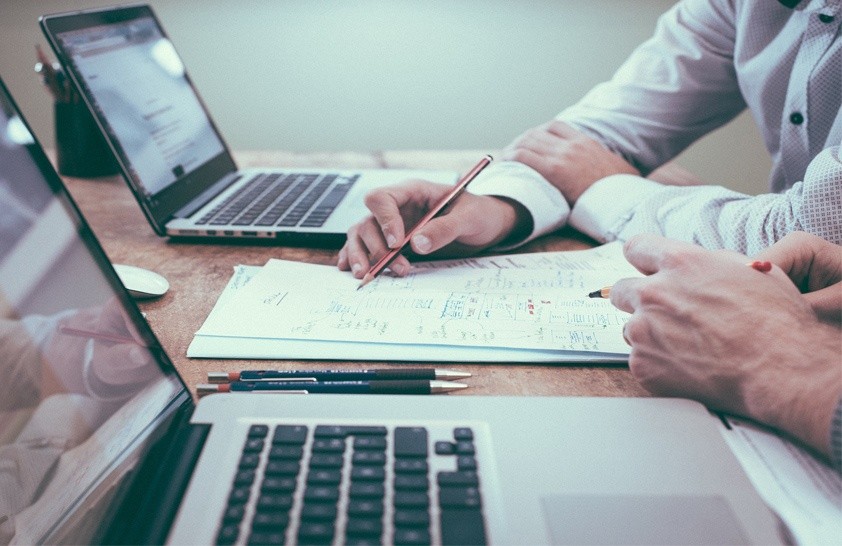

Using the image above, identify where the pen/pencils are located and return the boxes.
[59,325,136,344]
[357,155,494,290]
[208,369,479,384]
[585,260,772,298]
[197,380,476,397]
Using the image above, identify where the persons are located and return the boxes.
[336,1,842,278]
[610,229,842,469]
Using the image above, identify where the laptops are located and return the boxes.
[0,76,789,546]
[39,3,458,246]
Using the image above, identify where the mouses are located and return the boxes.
[110,262,169,296]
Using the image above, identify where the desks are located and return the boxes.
[39,149,842,546]
